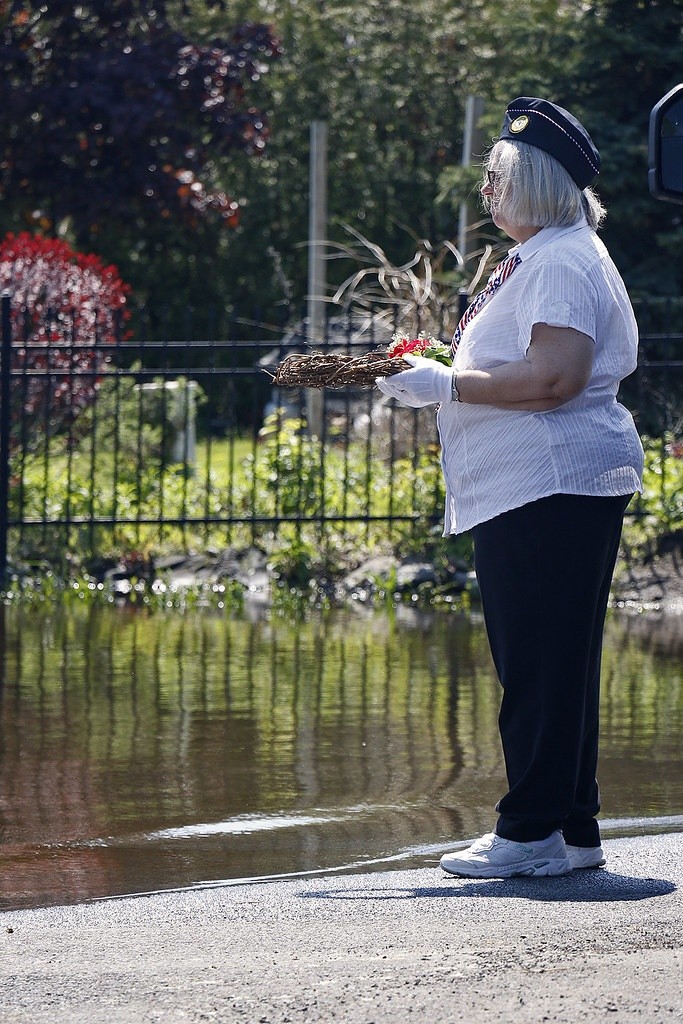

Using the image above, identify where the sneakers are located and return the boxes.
[439,828,606,877]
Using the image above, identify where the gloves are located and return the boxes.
[374,352,454,408]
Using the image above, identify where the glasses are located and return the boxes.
[483,166,505,184]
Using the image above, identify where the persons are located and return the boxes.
[377,115,640,879]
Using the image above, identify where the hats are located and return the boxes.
[499,97,603,191]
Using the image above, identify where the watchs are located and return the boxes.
[451,373,462,404]
